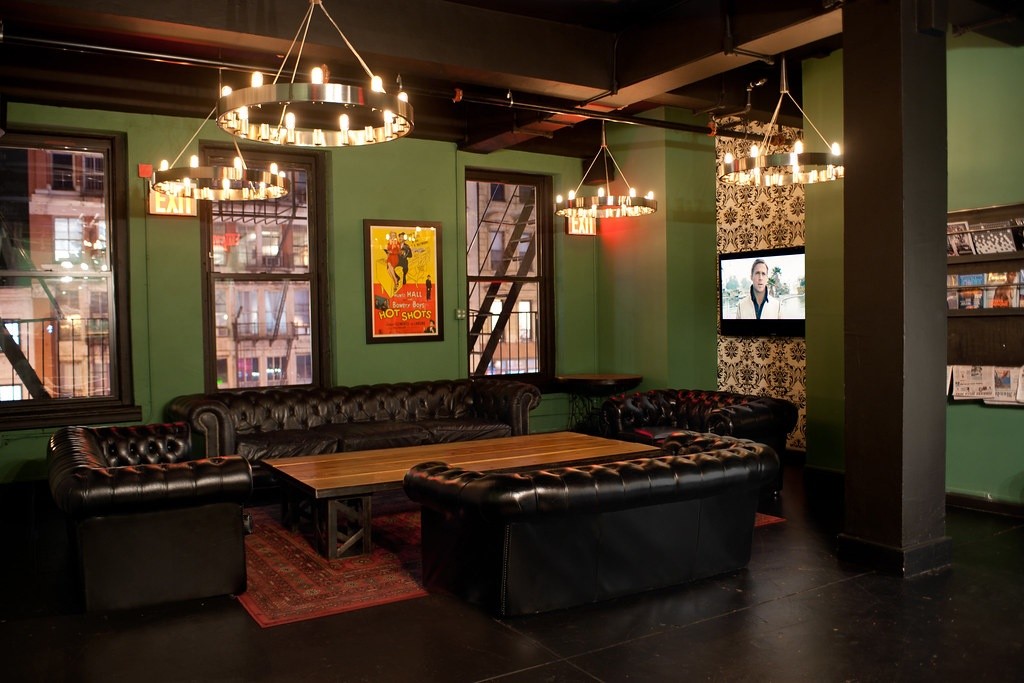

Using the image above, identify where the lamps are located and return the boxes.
[550,119,657,218]
[714,51,844,188]
[214,0,416,150]
[146,49,291,200]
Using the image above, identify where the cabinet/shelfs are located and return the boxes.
[943,203,1024,410]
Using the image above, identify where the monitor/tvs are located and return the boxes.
[719,246,805,338]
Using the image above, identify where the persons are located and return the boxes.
[736,259,780,319]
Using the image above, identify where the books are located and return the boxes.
[946,218,1024,309]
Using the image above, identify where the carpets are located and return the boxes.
[232,489,784,628]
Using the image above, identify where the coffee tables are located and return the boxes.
[260,432,663,564]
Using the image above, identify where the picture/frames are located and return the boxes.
[362,218,445,345]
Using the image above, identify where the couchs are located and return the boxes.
[48,416,253,618]
[403,430,780,618]
[163,375,544,509]
[596,388,798,503]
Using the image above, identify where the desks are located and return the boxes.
[553,373,643,429]
